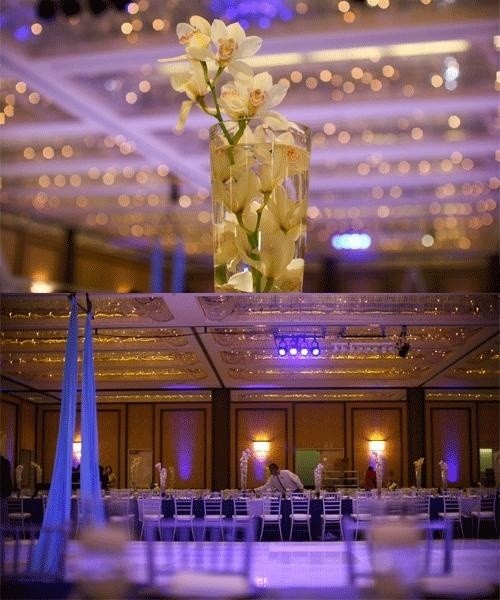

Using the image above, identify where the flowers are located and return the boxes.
[155,13,309,294]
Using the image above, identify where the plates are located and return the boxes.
[155,572,253,600]
[418,576,497,598]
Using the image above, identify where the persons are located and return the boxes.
[247,464,306,496]
[1,453,14,576]
[66,464,120,489]
[12,448,46,499]
[364,466,378,491]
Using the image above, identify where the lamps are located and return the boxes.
[273,332,326,358]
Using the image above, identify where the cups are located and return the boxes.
[367,521,422,599]
[80,523,130,600]
